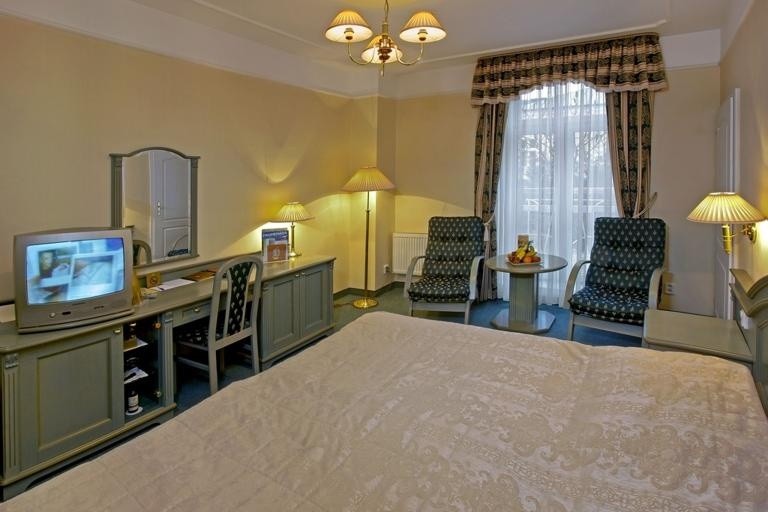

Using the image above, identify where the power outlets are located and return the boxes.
[384,264,390,277]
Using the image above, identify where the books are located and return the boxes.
[261,227,290,263]
[185,266,223,281]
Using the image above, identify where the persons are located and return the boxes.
[36,249,55,277]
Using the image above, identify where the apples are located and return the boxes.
[523,256,531,263]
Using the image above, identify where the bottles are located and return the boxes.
[128,383,139,413]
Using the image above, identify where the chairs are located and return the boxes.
[563,215,669,351]
[403,215,491,326]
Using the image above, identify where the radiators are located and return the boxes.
[388,231,431,278]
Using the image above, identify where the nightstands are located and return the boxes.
[640,306,752,366]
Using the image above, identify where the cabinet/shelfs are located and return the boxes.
[0,251,336,502]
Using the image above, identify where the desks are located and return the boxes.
[484,252,568,336]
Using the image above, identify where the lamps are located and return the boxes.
[322,1,448,77]
[338,164,395,312]
[275,203,314,260]
[683,191,766,257]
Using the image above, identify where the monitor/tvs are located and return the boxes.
[13,227,136,334]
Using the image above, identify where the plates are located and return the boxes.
[504,252,542,266]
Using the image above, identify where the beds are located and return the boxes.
[1,266,768,511]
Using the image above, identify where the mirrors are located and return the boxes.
[108,142,201,270]
